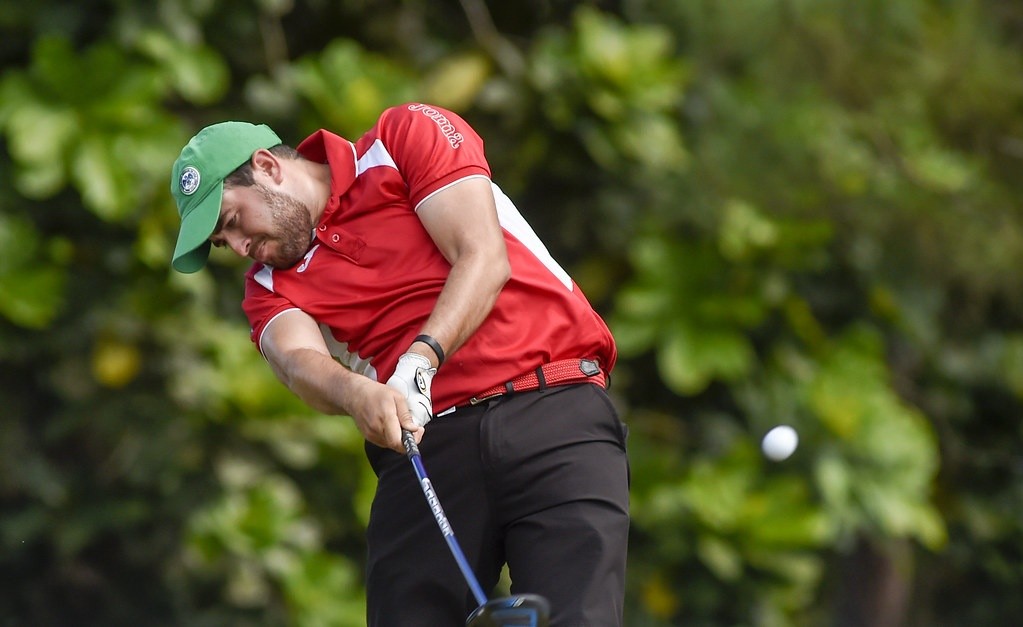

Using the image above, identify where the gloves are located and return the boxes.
[386,351,431,427]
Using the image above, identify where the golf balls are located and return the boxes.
[761,425,799,462]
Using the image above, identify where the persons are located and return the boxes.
[170,102,631,627]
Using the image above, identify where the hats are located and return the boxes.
[170,121,283,274]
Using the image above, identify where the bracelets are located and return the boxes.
[411,335,445,369]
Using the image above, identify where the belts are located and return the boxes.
[431,357,607,417]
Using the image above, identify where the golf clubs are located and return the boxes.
[402,428,551,627]
